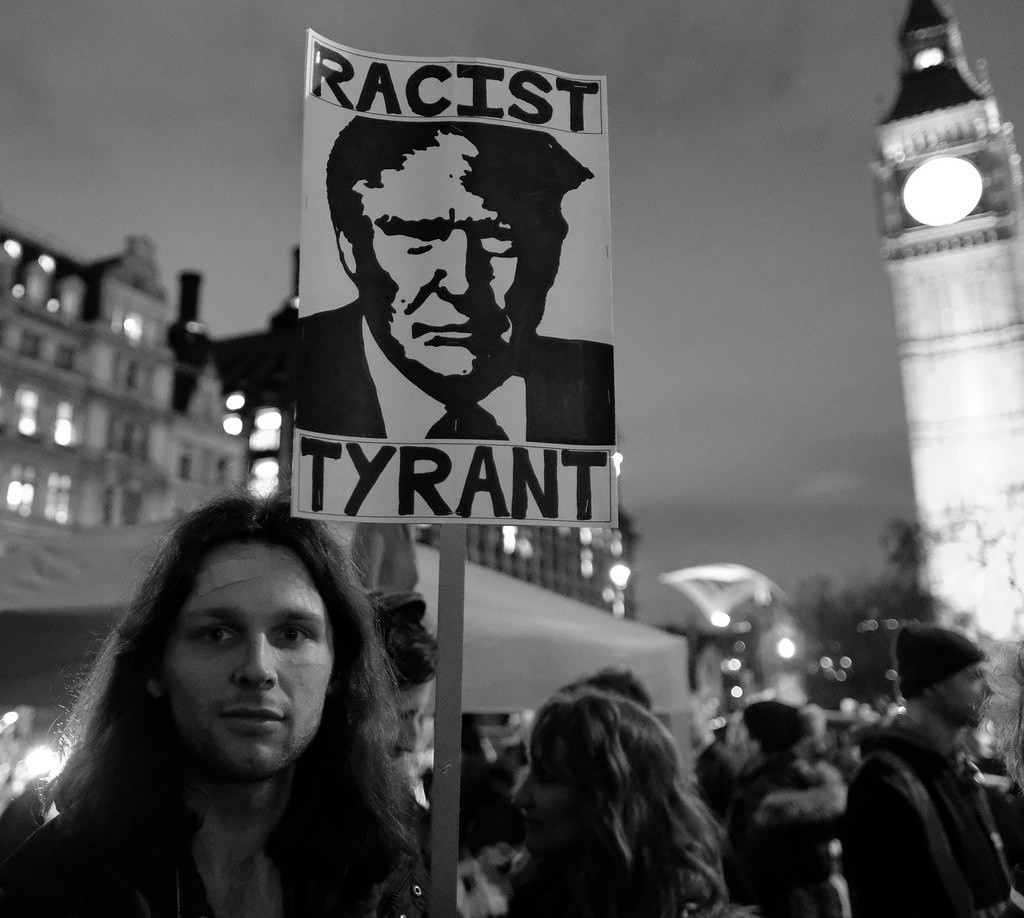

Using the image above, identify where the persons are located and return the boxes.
[0,493,1024,918]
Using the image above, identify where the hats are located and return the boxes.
[744,700,809,755]
[897,626,988,699]
